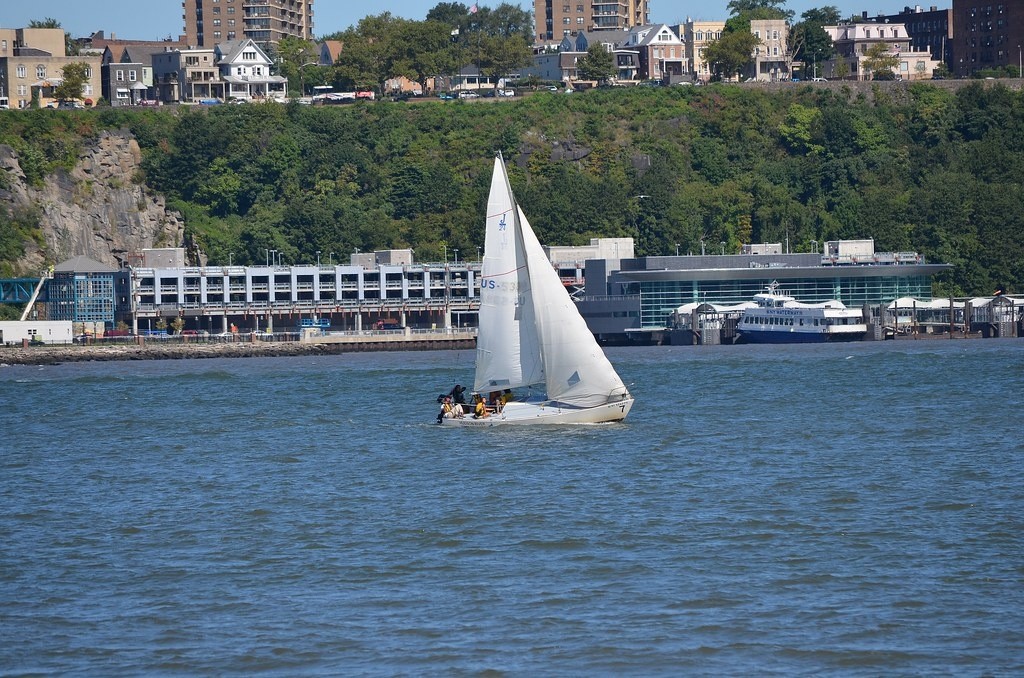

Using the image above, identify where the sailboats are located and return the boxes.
[435,152,632,432]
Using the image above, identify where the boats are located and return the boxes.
[734,289,868,342]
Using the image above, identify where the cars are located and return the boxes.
[0,77,828,103]
[30,317,427,346]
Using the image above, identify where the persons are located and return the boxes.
[452,322,457,332]
[492,396,505,415]
[440,397,466,419]
[103,329,109,343]
[469,388,483,413]
[473,396,490,419]
[231,323,239,337]
[436,397,446,424]
[452,385,470,414]
[486,380,502,413]
[499,388,513,406]
